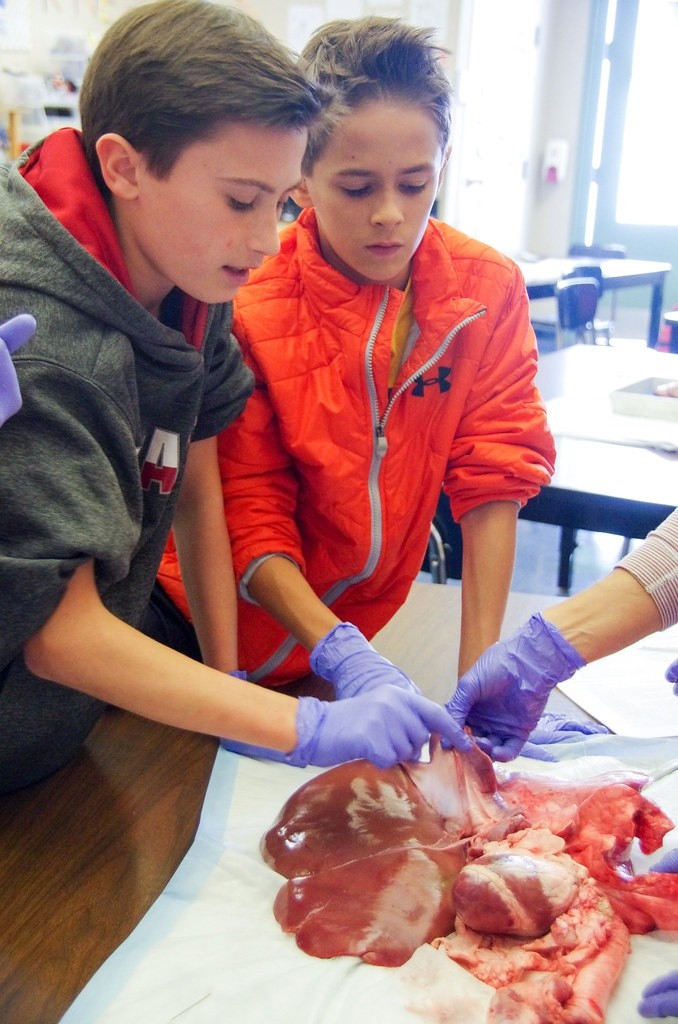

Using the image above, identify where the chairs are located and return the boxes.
[554,243,628,351]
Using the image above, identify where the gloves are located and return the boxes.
[285,694,466,773]
[220,671,309,768]
[648,848,677,873]
[309,622,422,701]
[441,614,586,763]
[518,711,608,762]
[638,968,678,1019]
[0,313,36,428]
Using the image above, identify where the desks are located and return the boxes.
[517,343,678,598]
[513,254,672,353]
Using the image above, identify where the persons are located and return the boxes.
[438,504,678,763]
[133,16,556,763]
[0,0,475,796]
